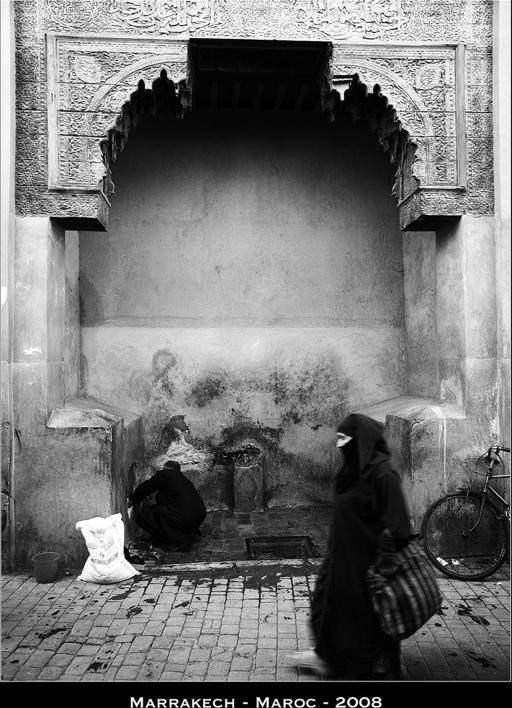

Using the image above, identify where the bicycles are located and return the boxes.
[420,443,511,583]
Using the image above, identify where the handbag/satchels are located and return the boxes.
[364,532,443,639]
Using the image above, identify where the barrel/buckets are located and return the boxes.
[32,552,60,583]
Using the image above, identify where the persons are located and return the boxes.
[285,413,413,682]
[128,460,208,550]
